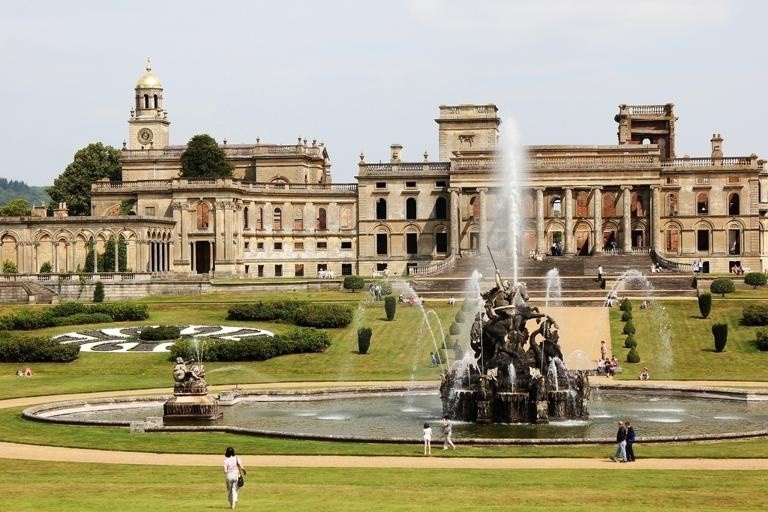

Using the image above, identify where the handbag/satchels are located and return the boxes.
[238,477,243,487]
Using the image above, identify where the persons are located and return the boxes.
[550,241,564,256]
[398,294,403,304]
[223,447,247,510]
[650,262,664,274]
[610,420,636,462]
[419,296,424,305]
[441,417,456,451]
[371,266,376,279]
[422,422,433,455]
[449,296,455,305]
[530,249,543,262]
[639,367,649,381]
[384,267,390,279]
[174,357,201,380]
[16,368,32,376]
[369,283,382,302]
[732,265,745,275]
[408,295,414,306]
[597,340,619,378]
[598,265,603,281]
[692,258,703,277]
[318,268,333,279]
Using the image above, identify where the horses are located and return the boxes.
[483,281,560,361]
